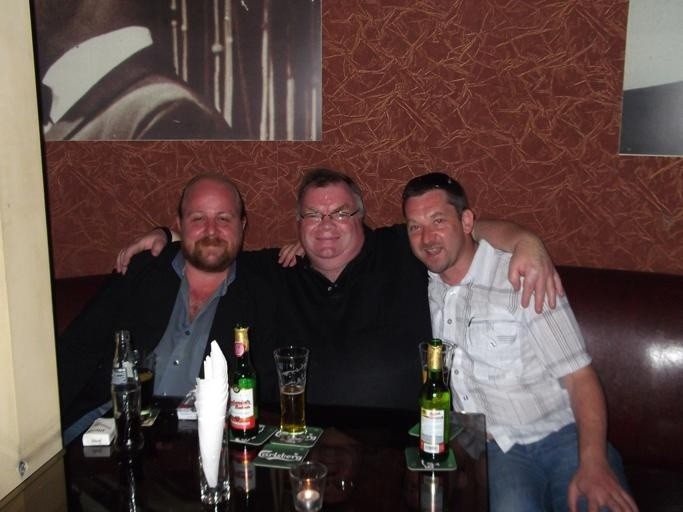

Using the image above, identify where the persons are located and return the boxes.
[114,168,566,412]
[277,171,643,512]
[54,173,249,447]
[34,2,234,138]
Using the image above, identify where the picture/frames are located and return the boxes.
[2,390,493,512]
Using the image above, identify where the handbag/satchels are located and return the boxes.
[153,224,172,243]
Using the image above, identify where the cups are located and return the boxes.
[300,208,358,222]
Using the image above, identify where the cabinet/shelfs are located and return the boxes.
[226,320,259,440]
[115,452,141,512]
[109,327,145,452]
[233,443,257,512]
[418,337,453,463]
[417,472,443,512]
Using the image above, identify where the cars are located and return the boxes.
[272,344,310,444]
[289,461,328,512]
[129,344,156,424]
[194,436,232,507]
[419,340,452,385]
